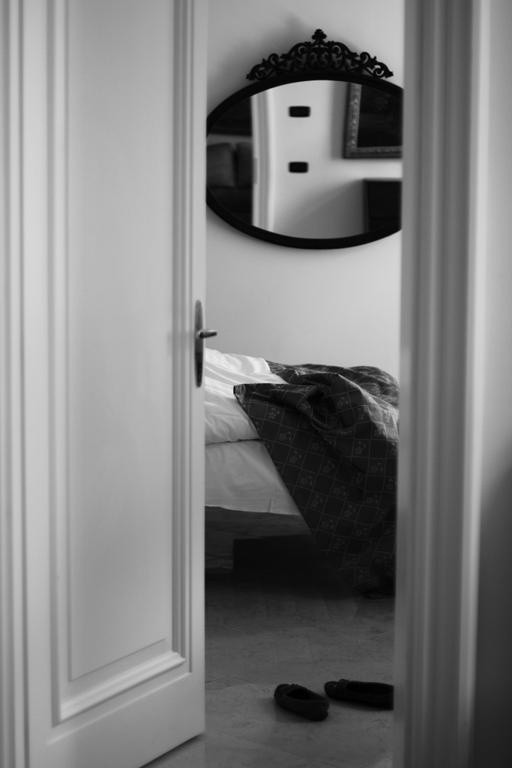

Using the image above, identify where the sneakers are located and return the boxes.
[325,679,393,712]
[275,682,329,721]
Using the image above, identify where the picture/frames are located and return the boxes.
[362,177,403,234]
[344,81,404,159]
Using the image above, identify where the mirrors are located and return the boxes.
[204,29,403,249]
